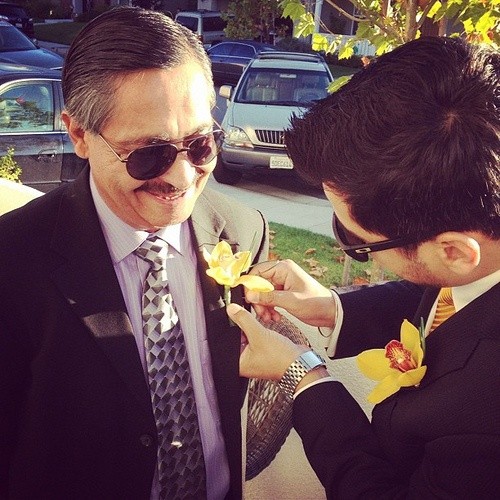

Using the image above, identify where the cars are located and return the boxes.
[0,3,89,193]
[205,40,282,86]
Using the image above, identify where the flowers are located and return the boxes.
[203,238,273,323]
[353,316,432,404]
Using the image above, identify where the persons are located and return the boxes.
[227,35,500,500]
[8,86,47,129]
[0,6,269,500]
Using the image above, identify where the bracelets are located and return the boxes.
[278,351,327,399]
[318,290,338,338]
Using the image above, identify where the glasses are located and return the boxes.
[97,118,229,181]
[331,210,454,263]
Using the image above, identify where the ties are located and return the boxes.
[428,286,457,338]
[136,237,210,500]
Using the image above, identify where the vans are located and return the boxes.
[174,8,237,50]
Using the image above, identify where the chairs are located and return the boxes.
[294,73,326,102]
[247,72,279,102]
[247,309,314,476]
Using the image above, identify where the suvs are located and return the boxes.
[212,49,336,186]
